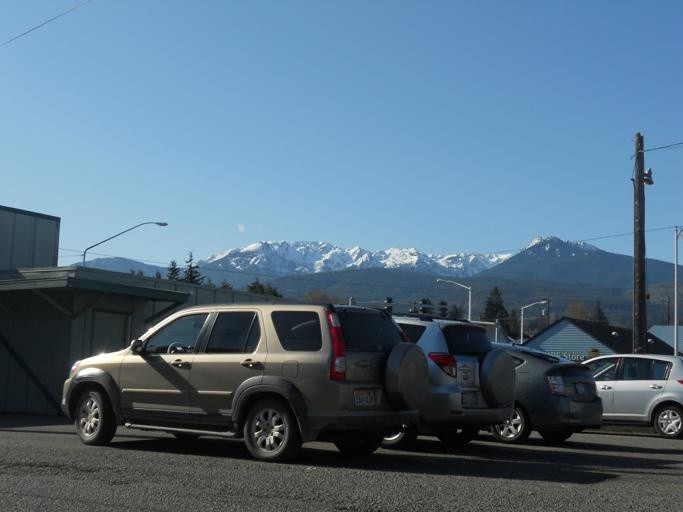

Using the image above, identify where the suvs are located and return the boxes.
[574,353,682,441]
[378,310,519,452]
[61,299,435,465]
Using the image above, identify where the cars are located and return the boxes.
[485,340,605,447]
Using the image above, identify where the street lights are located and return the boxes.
[435,278,473,323]
[519,301,550,344]
